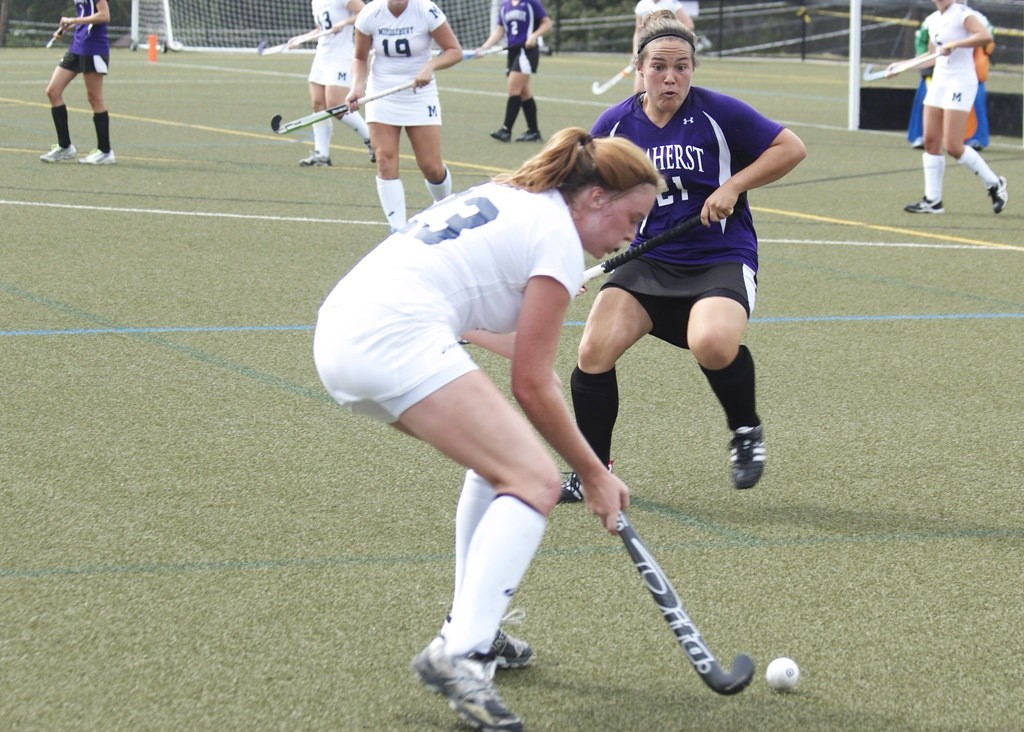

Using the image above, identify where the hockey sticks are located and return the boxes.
[613,509,754,697]
[861,52,940,83]
[578,208,710,284]
[45,23,69,51]
[591,59,635,96]
[463,42,526,59]
[257,28,333,57]
[270,80,415,136]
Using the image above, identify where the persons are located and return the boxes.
[313,127,659,732]
[287,0,376,166]
[474,0,551,142]
[558,0,807,503]
[885,0,1008,214]
[344,0,463,232]
[40,0,116,165]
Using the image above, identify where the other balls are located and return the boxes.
[765,657,800,691]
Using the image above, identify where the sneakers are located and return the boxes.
[40,144,77,163]
[78,149,117,164]
[299,150,332,166]
[515,130,540,142]
[904,196,944,214]
[726,421,768,489]
[363,138,376,162]
[490,126,511,142]
[557,459,614,502]
[411,612,534,732]
[986,176,1008,214]
